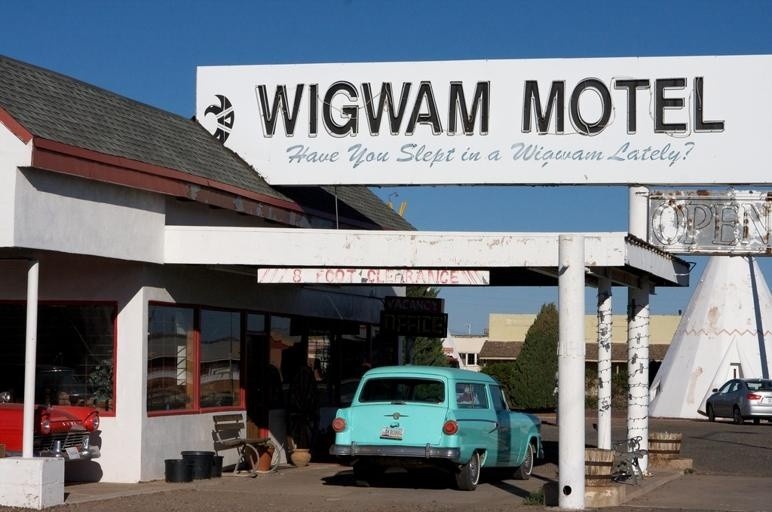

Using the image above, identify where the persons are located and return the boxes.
[457,384,480,405]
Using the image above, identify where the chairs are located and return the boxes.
[212,413,282,478]
[735,383,772,391]
[611,436,649,487]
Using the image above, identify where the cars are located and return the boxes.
[706,378,772,424]
[1,386,102,464]
[328,364,544,490]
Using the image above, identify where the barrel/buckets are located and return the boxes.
[180,450,215,478]
[212,455,224,477]
[164,458,195,484]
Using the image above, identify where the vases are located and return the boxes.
[163,451,224,483]
[290,448,312,468]
[245,443,276,471]
